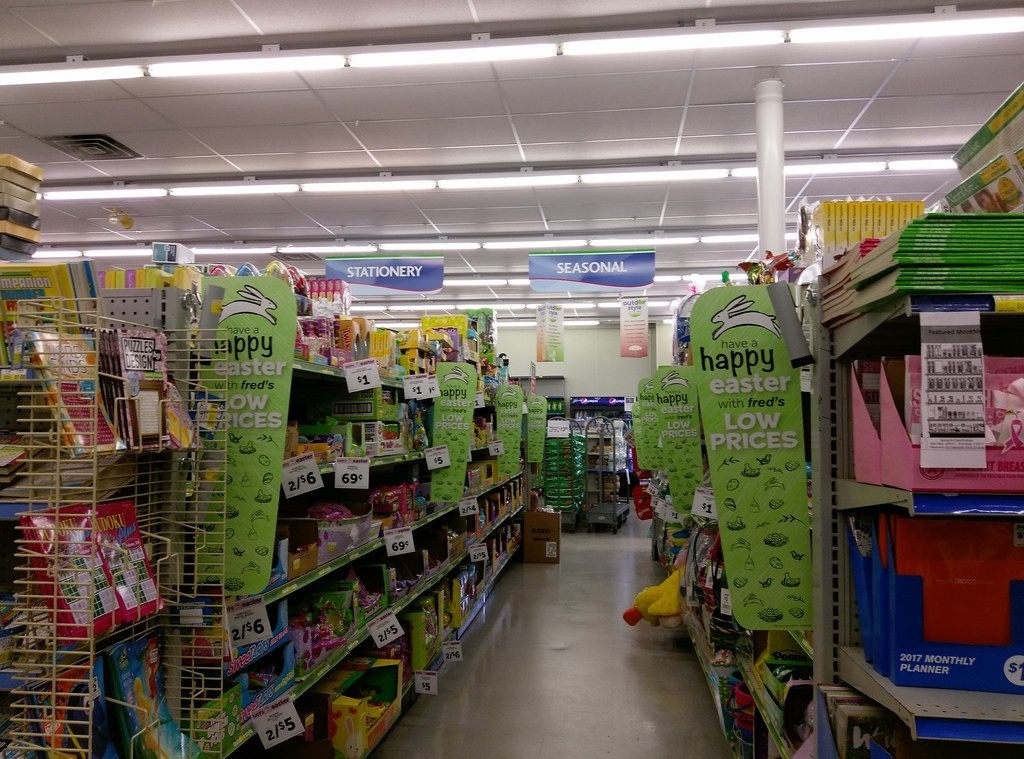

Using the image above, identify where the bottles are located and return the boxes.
[574,409,623,421]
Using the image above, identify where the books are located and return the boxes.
[821,211,1024,325]
[0,255,201,759]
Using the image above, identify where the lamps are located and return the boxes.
[351,273,744,329]
[35,154,958,201]
[34,230,797,260]
[0,7,1024,86]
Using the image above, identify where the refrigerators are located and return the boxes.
[568,395,640,499]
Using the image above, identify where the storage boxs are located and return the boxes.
[811,201,926,262]
[176,305,523,759]
[525,509,561,563]
[846,352,1024,696]
[751,628,814,753]
[0,153,352,314]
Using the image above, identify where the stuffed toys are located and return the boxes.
[623,565,690,628]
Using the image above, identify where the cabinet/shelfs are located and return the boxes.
[0,285,533,759]
[651,276,1024,759]
[536,395,635,534]
